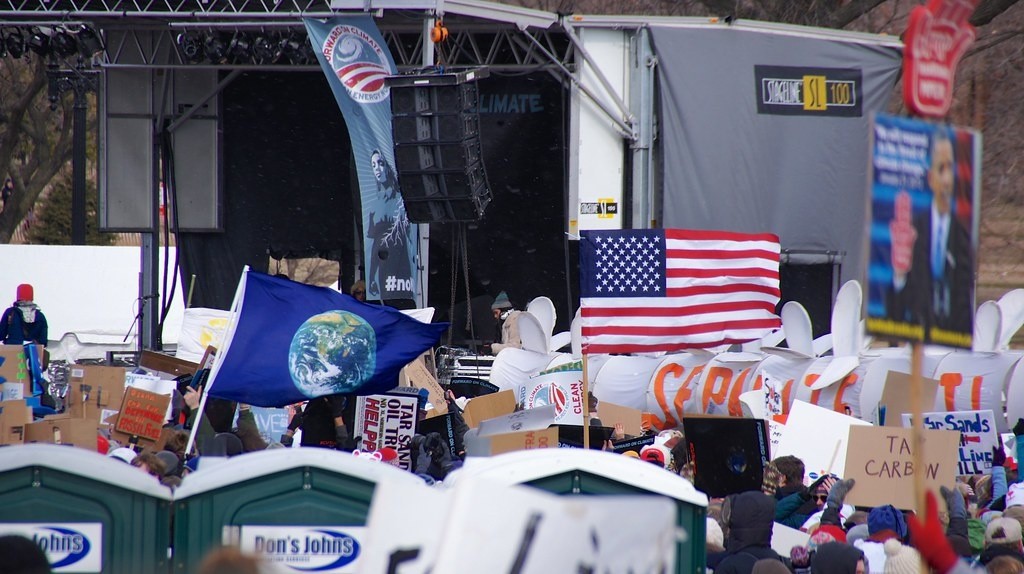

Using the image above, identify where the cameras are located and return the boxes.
[412,432,444,461]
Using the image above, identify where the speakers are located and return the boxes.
[389,80,495,223]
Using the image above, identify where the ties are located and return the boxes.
[933,217,946,278]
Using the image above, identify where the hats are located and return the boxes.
[156,451,180,475]
[814,473,840,493]
[884,538,930,574]
[975,475,992,499]
[706,517,726,553]
[491,291,512,309]
[17,284,33,300]
[721,493,773,552]
[110,447,137,464]
[641,448,664,464]
[182,457,198,472]
[621,451,638,458]
[763,461,780,495]
[806,531,836,553]
[350,280,366,296]
[845,524,870,547]
[985,518,1023,544]
[1006,482,1024,508]
[868,506,908,537]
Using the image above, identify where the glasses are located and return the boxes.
[355,289,365,296]
[813,495,827,503]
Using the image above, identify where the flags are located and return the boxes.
[205,267,452,409]
[301,15,422,300]
[579,228,785,355]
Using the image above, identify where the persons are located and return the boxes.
[2,178,13,209]
[0,284,49,347]
[0,536,51,574]
[586,391,1024,574]
[98,382,481,498]
[478,291,528,356]
[886,127,974,335]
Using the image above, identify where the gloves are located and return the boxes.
[344,433,362,452]
[416,444,431,473]
[992,446,1006,466]
[940,486,968,519]
[808,474,828,493]
[1013,418,1024,436]
[790,546,808,568]
[287,412,303,432]
[172,389,182,409]
[438,442,451,463]
[587,392,598,412]
[907,491,958,574]
[827,479,856,512]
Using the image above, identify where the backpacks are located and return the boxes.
[738,550,792,574]
